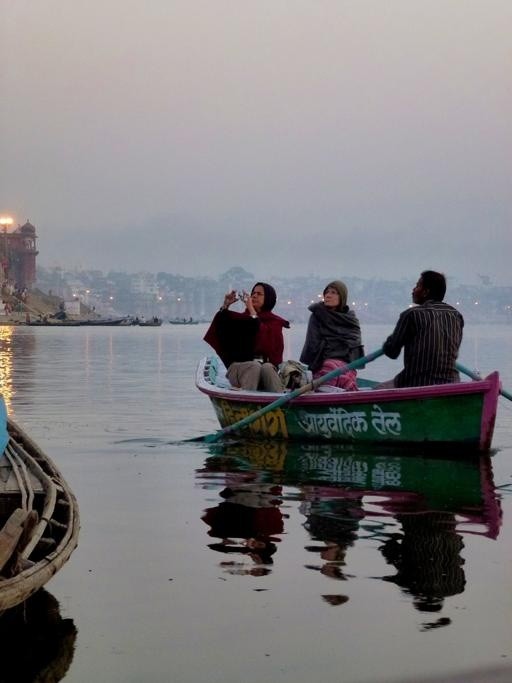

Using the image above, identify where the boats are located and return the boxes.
[21,315,202,327]
[189,440,504,543]
[194,350,504,457]
[0,407,85,618]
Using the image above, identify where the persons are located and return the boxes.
[300,280,367,393]
[376,491,468,638]
[204,280,286,393]
[376,268,464,387]
[298,481,365,605]
[195,466,289,594]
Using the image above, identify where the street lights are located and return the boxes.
[0,217,15,279]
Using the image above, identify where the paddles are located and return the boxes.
[181,349,384,443]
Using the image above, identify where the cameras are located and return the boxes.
[234,292,245,301]
